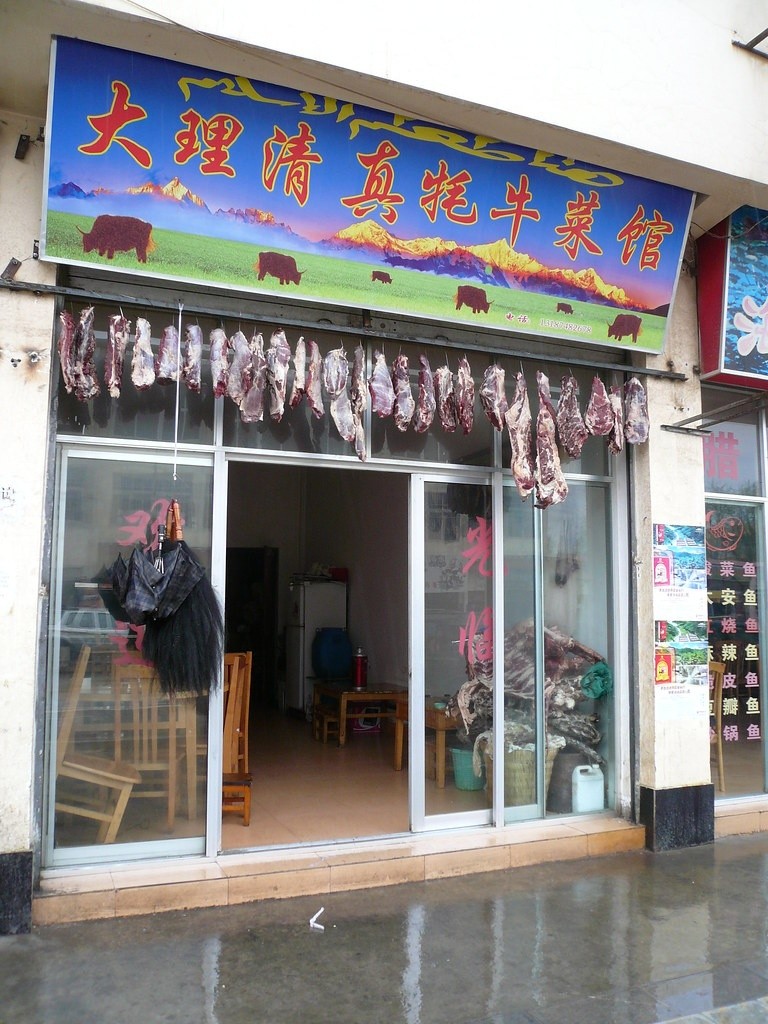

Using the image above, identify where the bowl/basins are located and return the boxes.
[434,702,446,710]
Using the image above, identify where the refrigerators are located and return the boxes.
[284,579,347,711]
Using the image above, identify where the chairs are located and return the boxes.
[54,642,252,845]
[710,634,760,792]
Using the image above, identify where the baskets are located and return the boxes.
[480,739,558,807]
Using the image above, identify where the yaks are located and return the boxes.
[455,286,494,315]
[607,314,643,343]
[253,252,306,285]
[557,303,575,315]
[372,271,393,284]
[75,214,152,264]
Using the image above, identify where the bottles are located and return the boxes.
[571,763,605,812]
[652,524,674,587]
[654,621,675,685]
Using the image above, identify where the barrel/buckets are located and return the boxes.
[312,626,353,680]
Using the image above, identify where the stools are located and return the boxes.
[425,735,450,780]
[315,712,347,744]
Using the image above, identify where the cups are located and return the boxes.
[79,678,92,694]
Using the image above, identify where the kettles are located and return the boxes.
[352,646,371,691]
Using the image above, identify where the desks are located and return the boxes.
[77,644,140,677]
[313,683,430,748]
[58,677,229,819]
[393,696,460,788]
